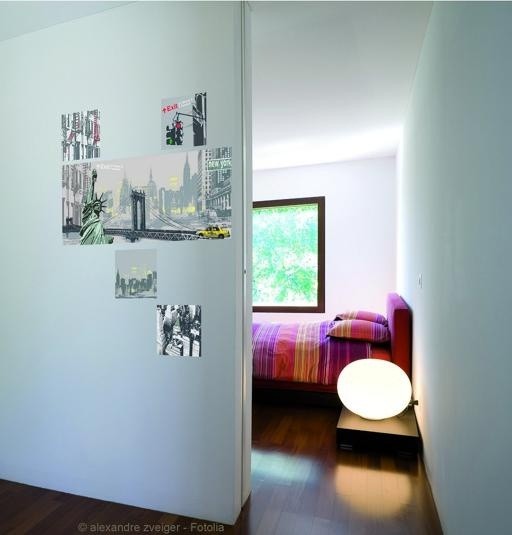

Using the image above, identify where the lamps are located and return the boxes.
[337,358,412,421]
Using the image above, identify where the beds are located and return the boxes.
[252,292,411,393]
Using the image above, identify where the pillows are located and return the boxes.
[325,319,391,343]
[336,311,387,326]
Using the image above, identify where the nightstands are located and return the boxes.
[336,407,420,464]
